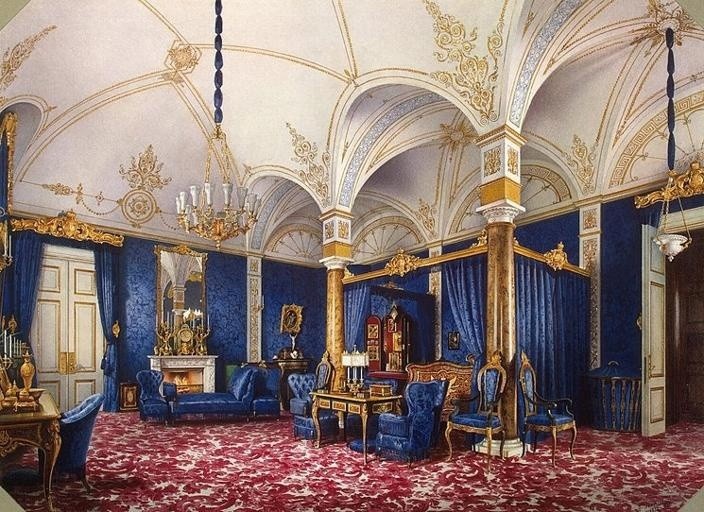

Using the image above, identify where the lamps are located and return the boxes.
[651,17,692,262]
[174,0,264,251]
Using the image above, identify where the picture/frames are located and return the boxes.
[447,331,460,350]
[279,304,303,335]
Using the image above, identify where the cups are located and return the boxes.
[289,353,298,359]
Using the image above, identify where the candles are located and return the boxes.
[4,329,21,360]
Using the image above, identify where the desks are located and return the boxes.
[0,385,59,510]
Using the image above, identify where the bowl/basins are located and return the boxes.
[16,387,46,400]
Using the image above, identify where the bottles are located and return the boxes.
[19,350,36,390]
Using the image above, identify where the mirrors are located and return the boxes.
[156,243,209,345]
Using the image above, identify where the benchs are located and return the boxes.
[405,360,474,423]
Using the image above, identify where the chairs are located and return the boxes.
[515,351,578,468]
[442,350,510,471]
[287,350,449,469]
[38,393,105,493]
[136,360,282,426]
